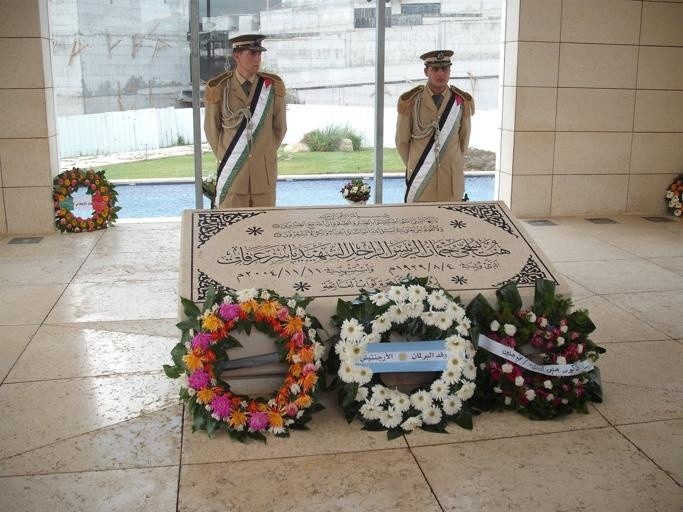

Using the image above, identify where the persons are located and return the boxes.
[202,33,288,209]
[393,49,476,203]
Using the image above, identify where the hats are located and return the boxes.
[227,34,267,53]
[419,49,456,69]
